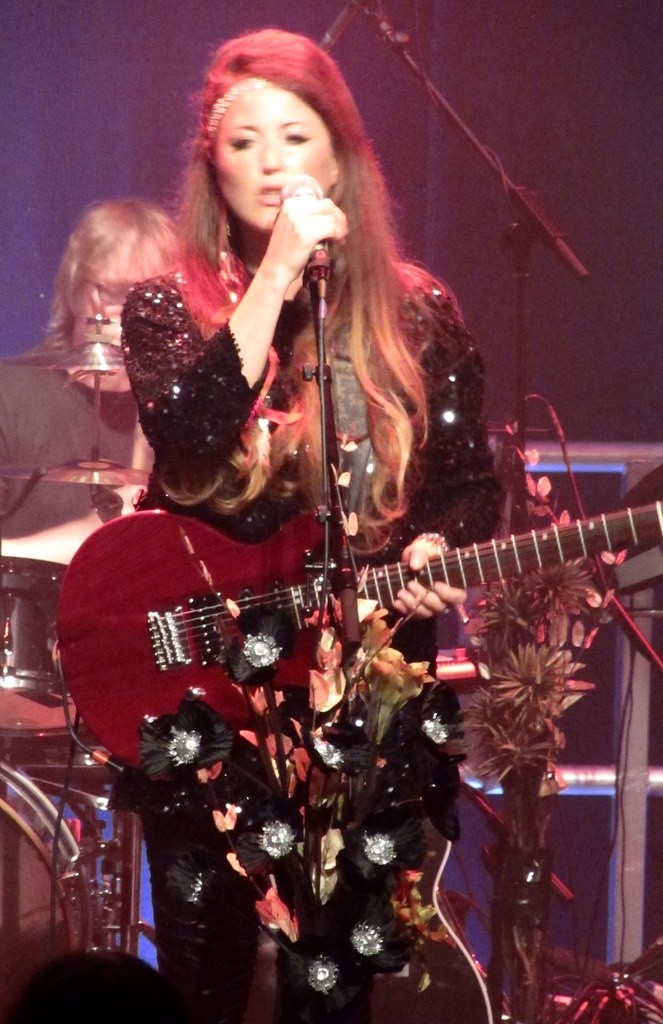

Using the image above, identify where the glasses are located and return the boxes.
[83,276,129,304]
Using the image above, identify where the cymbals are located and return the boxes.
[1,462,142,485]
[0,342,134,370]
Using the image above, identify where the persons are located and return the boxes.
[0,191,179,971]
[119,27,507,1024]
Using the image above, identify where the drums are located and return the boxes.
[0,763,102,1015]
[0,553,80,741]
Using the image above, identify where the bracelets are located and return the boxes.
[417,532,449,552]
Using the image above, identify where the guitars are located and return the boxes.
[56,503,663,780]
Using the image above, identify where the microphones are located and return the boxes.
[280,176,331,285]
[496,418,522,487]
[319,0,365,52]
